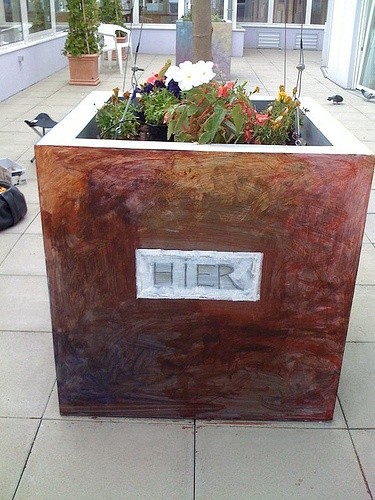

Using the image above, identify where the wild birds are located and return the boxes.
[327,94,344,105]
[360,88,375,102]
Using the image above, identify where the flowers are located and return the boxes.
[96,59,310,145]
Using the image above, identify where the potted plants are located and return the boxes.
[173,10,232,79]
[61,0,101,86]
[98,0,128,61]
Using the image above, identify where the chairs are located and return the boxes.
[96,25,133,75]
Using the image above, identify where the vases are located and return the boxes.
[33,89,375,423]
